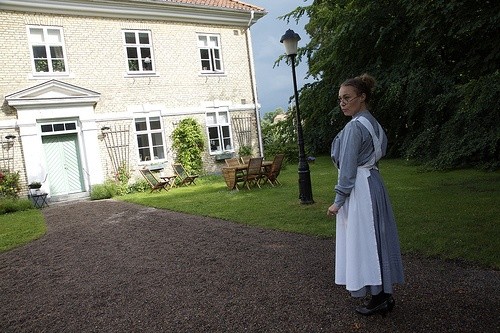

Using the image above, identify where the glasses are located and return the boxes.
[336,96,359,105]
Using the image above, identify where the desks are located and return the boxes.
[32,194,49,208]
[223,161,274,191]
[160,176,178,190]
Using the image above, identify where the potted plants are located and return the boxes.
[29,182,42,195]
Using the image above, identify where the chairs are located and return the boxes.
[139,169,169,193]
[171,164,199,187]
[225,154,285,191]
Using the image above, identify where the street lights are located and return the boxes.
[279,28,316,205]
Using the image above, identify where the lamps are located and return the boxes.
[101,127,110,137]
[5,134,16,144]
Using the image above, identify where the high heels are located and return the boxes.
[356,295,395,318]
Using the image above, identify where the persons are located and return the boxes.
[327,74,404,315]
